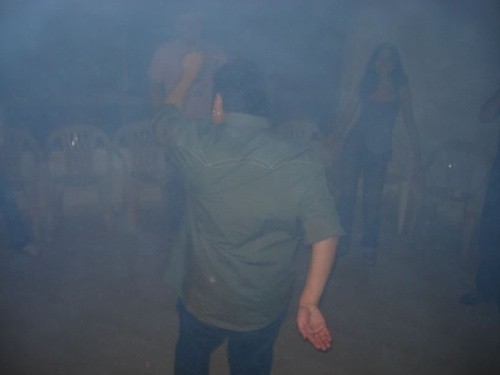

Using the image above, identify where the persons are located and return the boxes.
[1,176,46,260]
[150,52,348,374]
[140,5,226,235]
[329,43,425,268]
[459,86,499,307]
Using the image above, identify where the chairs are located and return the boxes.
[2,119,186,251]
[276,119,483,258]
